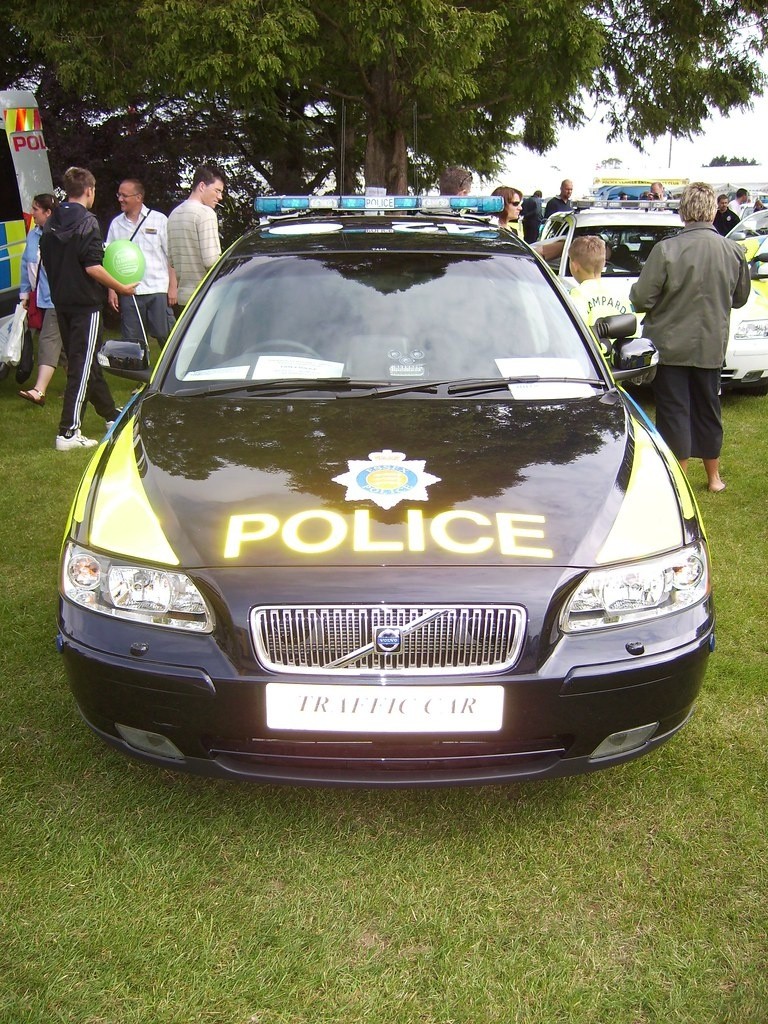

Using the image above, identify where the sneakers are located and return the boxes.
[105,406,124,431]
[55,428,98,450]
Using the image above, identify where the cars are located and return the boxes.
[53,194,718,793]
[483,176,767,397]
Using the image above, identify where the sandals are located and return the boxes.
[57,391,65,398]
[17,388,46,406]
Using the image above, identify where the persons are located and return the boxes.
[415,167,471,216]
[489,186,564,260]
[612,181,678,261]
[18,194,68,406]
[519,190,542,244]
[568,236,637,358]
[544,180,573,234]
[713,189,768,237]
[38,169,141,449]
[166,166,226,310]
[630,182,751,492]
[105,180,179,395]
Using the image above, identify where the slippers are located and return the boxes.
[716,479,728,493]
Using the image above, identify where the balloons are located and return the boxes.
[103,240,146,287]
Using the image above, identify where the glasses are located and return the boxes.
[507,200,521,207]
[208,185,225,195]
[116,192,141,197]
[460,171,473,187]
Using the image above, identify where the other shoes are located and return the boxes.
[130,382,143,394]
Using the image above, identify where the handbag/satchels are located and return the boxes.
[27,289,44,329]
[0,302,28,367]
[15,320,35,384]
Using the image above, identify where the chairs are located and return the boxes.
[252,277,522,359]
[611,240,655,268]
